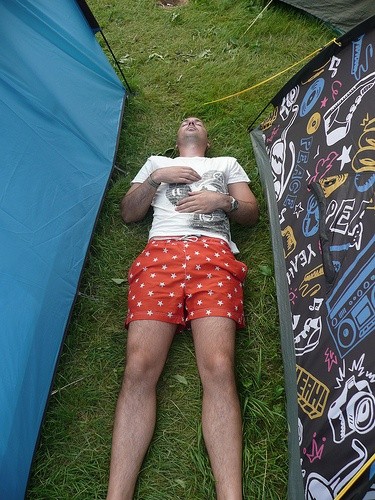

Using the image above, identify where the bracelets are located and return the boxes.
[148,175,161,189]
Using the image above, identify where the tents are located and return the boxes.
[0,0,132,500]
[249,16,375,500]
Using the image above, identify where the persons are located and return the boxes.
[106,117,260,500]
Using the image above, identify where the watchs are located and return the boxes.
[228,196,239,213]
